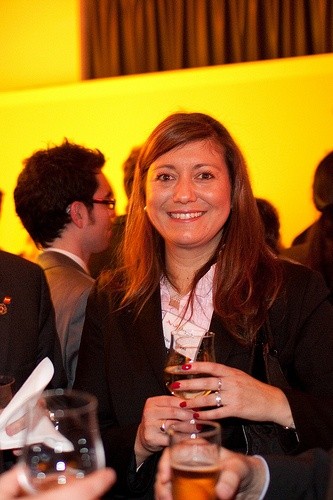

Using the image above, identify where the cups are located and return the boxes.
[166,420,222,500]
[167,330,215,400]
[17,389,104,494]
[0,375,17,410]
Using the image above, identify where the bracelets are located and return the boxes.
[290,423,296,429]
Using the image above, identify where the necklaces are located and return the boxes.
[165,274,182,309]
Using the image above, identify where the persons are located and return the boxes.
[63,112,333,500]
[0,249,66,472]
[13,137,117,390]
[87,147,143,276]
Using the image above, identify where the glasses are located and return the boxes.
[65,196,115,212]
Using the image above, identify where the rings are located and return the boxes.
[160,420,165,433]
[215,391,221,407]
[218,379,222,390]
